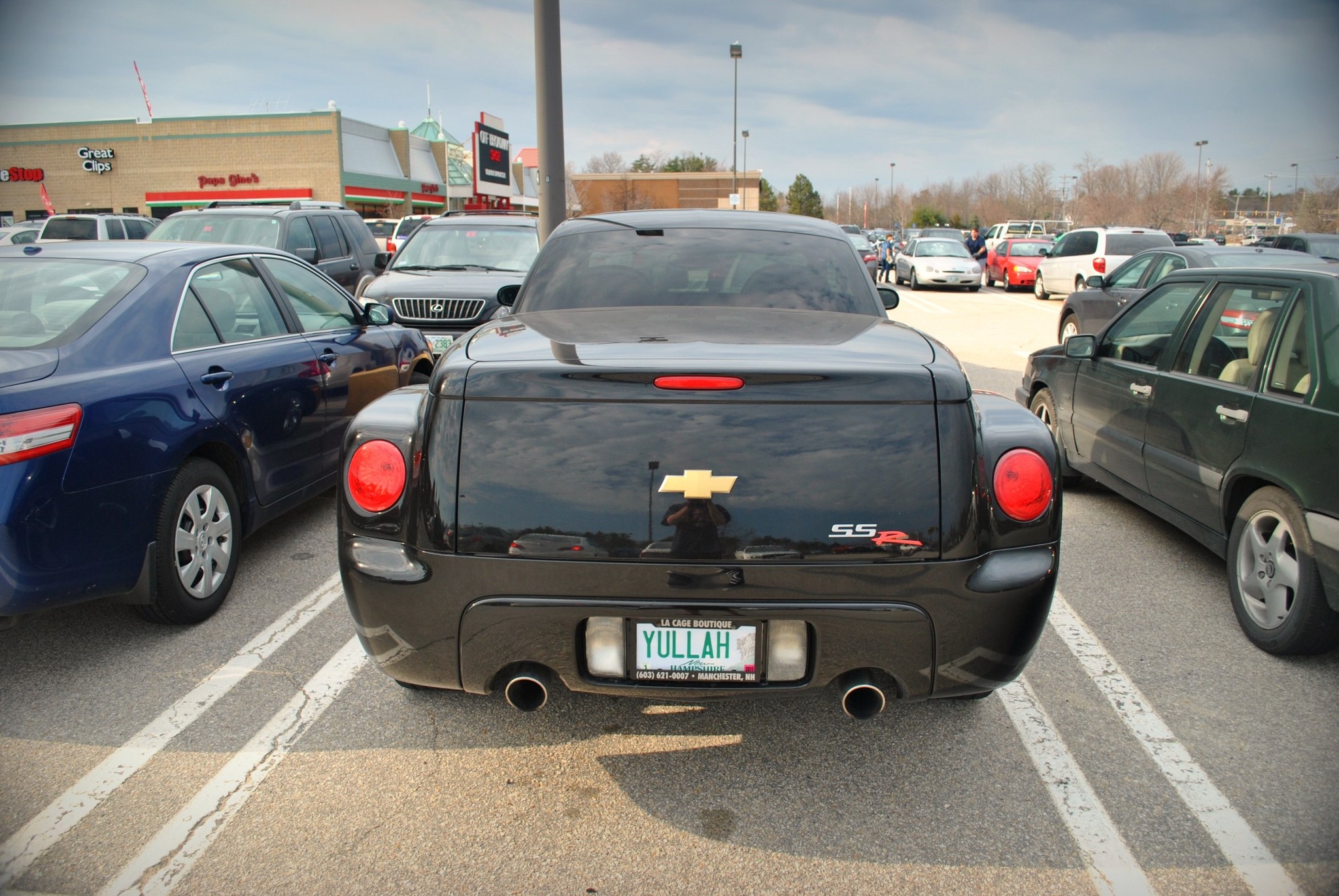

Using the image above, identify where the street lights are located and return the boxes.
[1073,176,1078,199]
[1291,163,1298,234]
[1192,140,1208,239]
[729,43,895,210]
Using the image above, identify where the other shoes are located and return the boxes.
[879,276,881,282]
[885,280,890,283]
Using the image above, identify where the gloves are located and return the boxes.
[973,253,980,257]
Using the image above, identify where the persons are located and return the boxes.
[965,226,988,288]
[878,233,893,282]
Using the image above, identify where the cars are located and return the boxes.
[2,218,435,625]
[1204,234,1226,245]
[1173,241,1204,247]
[336,208,1065,722]
[1188,238,1220,246]
[1057,245,1339,345]
[1168,233,1188,242]
[1240,234,1266,246]
[1016,267,1338,656]
[1271,234,1339,265]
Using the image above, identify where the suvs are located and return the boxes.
[11,213,157,241]
[144,199,382,297]
[131,213,161,225]
[838,225,1175,300]
[360,210,542,355]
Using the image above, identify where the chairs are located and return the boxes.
[567,265,655,298]
[21,236,33,243]
[45,286,96,304]
[173,287,253,351]
[1162,264,1186,277]
[1218,307,1310,394]
[740,263,831,295]
[0,311,47,336]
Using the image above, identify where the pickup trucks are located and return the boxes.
[983,217,1074,250]
